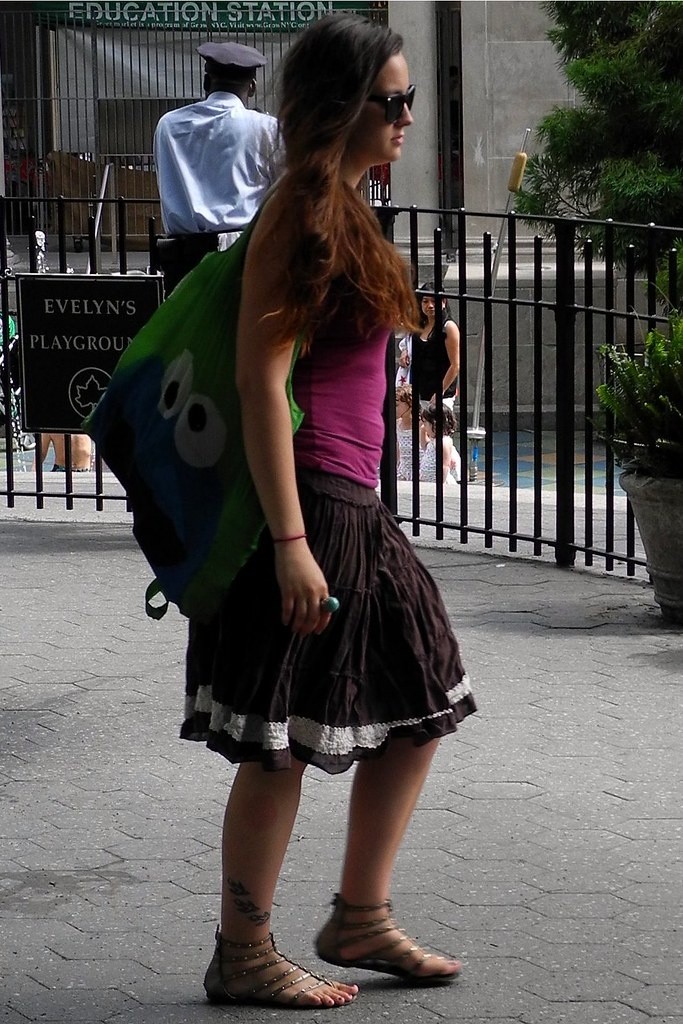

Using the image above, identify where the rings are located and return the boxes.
[320,597,339,613]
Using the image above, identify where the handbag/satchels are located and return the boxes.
[81,182,303,620]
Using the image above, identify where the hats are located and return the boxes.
[196,41,267,75]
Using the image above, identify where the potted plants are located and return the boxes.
[586,308,683,624]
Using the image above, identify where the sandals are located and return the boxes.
[315,892,461,982]
[203,925,357,1007]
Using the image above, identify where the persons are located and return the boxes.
[170,14,482,1010]
[151,42,288,237]
[395,283,468,485]
[30,432,92,471]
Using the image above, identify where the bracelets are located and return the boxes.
[271,534,308,543]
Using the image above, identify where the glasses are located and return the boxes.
[365,85,416,124]
